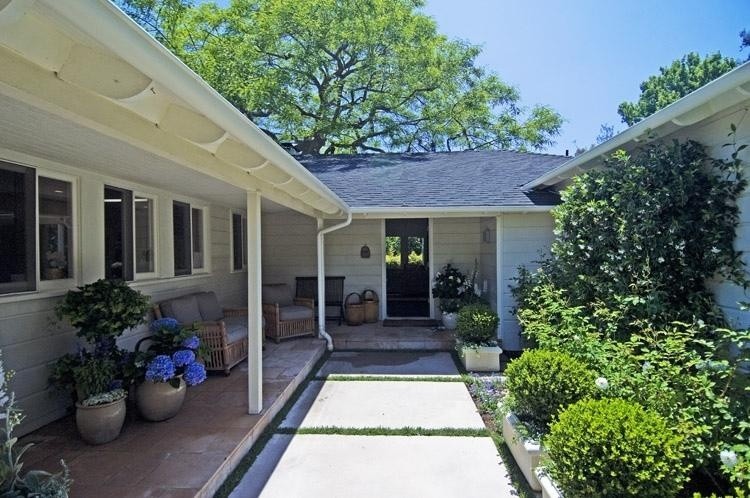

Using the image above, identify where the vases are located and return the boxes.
[73,392,128,444]
[443,309,460,331]
[132,375,188,422]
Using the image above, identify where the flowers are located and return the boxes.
[75,333,132,406]
[140,319,213,387]
[429,260,470,312]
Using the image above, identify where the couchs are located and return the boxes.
[262,282,316,343]
[151,291,265,377]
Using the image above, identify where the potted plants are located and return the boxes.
[530,398,691,498]
[452,300,503,372]
[496,346,602,488]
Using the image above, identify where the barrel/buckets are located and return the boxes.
[362,289,379,323]
[343,292,365,324]
